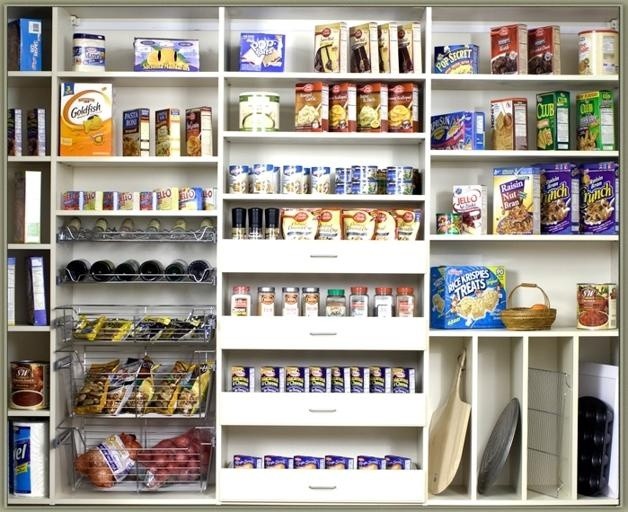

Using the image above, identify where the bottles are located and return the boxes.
[165,259,188,281]
[349,287,369,317]
[258,288,276,316]
[8,360,49,410]
[265,208,280,240]
[395,287,416,317]
[91,260,115,281]
[173,220,186,237]
[248,207,263,239]
[578,282,620,331]
[118,219,133,239]
[579,30,621,76]
[326,289,347,317]
[139,260,164,281]
[301,287,320,316]
[238,92,281,131]
[66,259,91,281]
[188,260,212,281]
[282,287,301,316]
[115,259,139,281]
[201,217,212,234]
[231,286,251,316]
[147,219,160,237]
[373,288,395,317]
[231,208,247,239]
[68,218,82,239]
[92,218,108,238]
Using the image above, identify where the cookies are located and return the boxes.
[82,115,103,133]
[494,112,512,148]
[147,48,189,70]
[580,129,600,150]
[455,291,500,319]
[537,127,553,149]
[433,294,446,315]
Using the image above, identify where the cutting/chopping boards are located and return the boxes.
[429,348,470,495]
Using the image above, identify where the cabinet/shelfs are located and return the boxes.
[1,1,626,507]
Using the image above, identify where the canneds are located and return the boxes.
[437,213,464,234]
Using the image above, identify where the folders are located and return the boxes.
[31,256,47,327]
